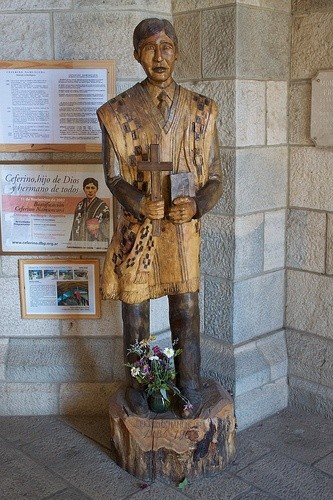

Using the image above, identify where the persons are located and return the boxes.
[96,18,222,419]
[69,178,109,249]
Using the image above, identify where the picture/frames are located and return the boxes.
[0,61,118,153]
[0,160,120,255]
[18,258,101,319]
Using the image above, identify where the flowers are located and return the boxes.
[121,330,195,414]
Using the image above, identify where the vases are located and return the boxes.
[149,390,173,414]
